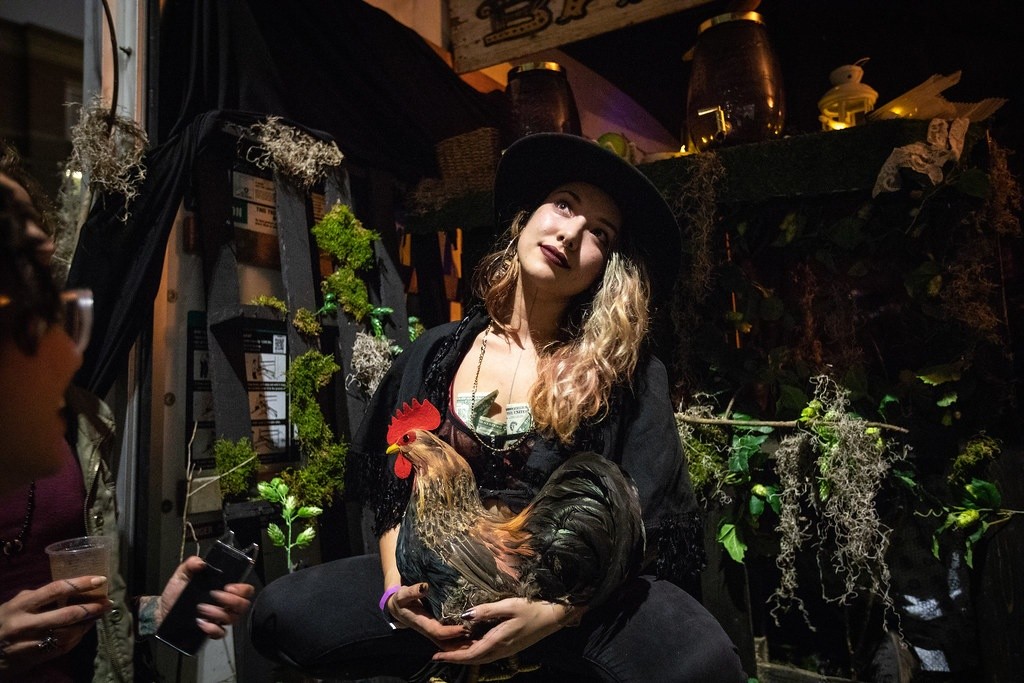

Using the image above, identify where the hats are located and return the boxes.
[493,133,682,299]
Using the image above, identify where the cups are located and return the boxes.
[45,536,113,622]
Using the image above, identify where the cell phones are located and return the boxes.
[155,541,255,657]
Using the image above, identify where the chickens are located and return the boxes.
[385,397,646,683]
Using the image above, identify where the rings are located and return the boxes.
[39,638,58,655]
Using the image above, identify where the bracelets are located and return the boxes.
[380,586,409,629]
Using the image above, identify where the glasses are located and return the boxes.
[0,288,95,355]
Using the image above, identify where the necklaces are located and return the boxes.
[470,320,534,451]
[0,481,35,554]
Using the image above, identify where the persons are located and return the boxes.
[249,134,747,683]
[0,139,253,683]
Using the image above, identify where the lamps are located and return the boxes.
[818,58,878,131]
